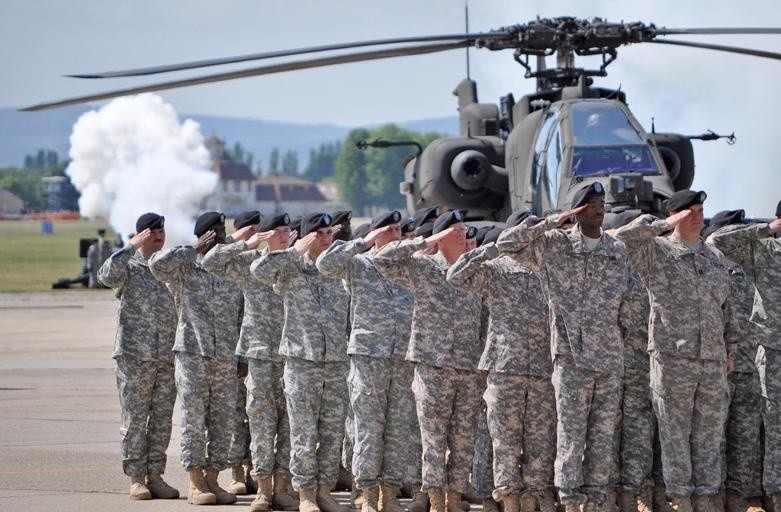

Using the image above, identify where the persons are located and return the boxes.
[93,211,183,501]
[146,180,780,512]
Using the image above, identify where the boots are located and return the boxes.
[132,467,152,499]
[245,467,781,512]
[146,468,179,499]
[201,466,237,503]
[184,468,215,505]
[228,467,247,494]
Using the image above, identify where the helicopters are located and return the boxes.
[18,13,781,219]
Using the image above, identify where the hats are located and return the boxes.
[571,181,606,210]
[136,212,165,235]
[709,209,745,231]
[234,205,542,237]
[194,212,225,236]
[666,189,707,213]
[613,212,644,230]
